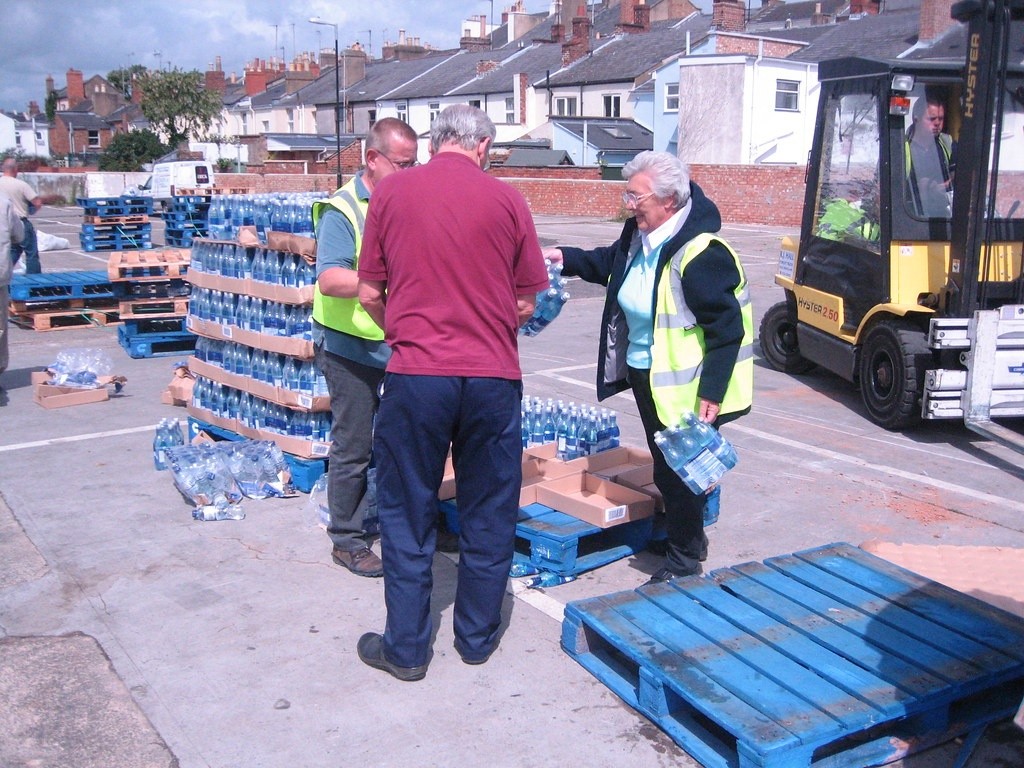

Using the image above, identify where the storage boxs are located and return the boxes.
[9,196,662,528]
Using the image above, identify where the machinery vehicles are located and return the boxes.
[755,1,1024,459]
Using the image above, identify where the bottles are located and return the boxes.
[153,191,381,536]
[526,571,577,588]
[516,258,571,339]
[509,563,540,577]
[519,395,620,462]
[653,413,738,495]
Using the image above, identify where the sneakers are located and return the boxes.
[332,546,383,577]
[357,632,426,680]
[453,639,491,664]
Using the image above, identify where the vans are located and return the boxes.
[134,160,215,198]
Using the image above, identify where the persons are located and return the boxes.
[312,116,417,574]
[0,158,41,273]
[874,95,957,199]
[357,105,548,682]
[0,192,25,375]
[542,150,755,588]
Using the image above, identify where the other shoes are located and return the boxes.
[646,537,707,560]
[640,563,703,586]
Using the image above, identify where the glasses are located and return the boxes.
[376,149,421,171]
[622,191,654,210]
[480,137,491,172]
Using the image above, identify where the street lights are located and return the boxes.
[308,18,342,190]
